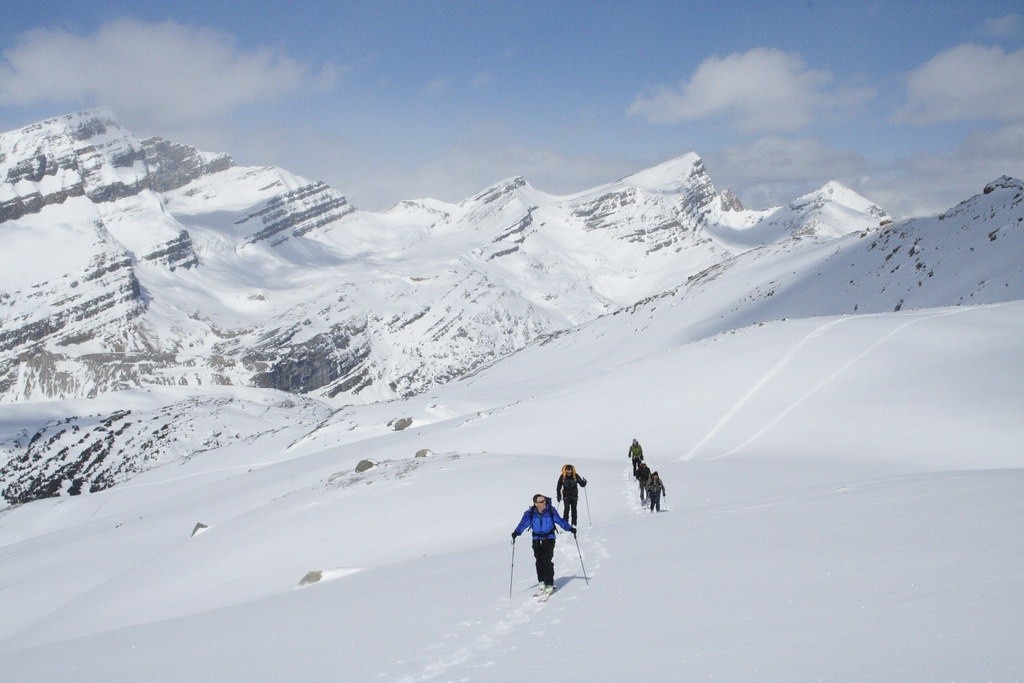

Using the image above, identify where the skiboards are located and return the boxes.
[531,581,556,604]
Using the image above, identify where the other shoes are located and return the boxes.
[544,585,553,594]
[539,581,545,590]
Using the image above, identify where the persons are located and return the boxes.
[645,471,666,512]
[511,493,577,595]
[636,459,651,506]
[556,464,587,529]
[628,438,644,476]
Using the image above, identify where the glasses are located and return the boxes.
[536,499,545,503]
[567,472,572,474]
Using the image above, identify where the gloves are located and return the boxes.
[511,532,517,538]
[570,528,576,533]
[557,497,561,502]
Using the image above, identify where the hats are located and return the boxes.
[654,472,658,476]
